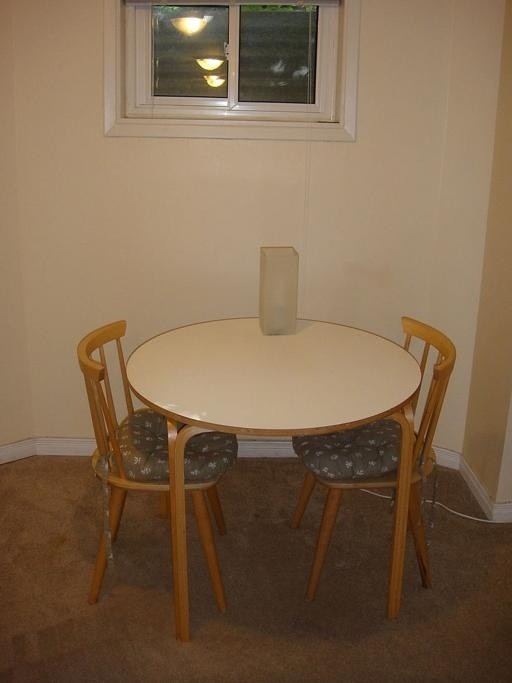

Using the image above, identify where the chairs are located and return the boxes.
[76,319,239,642]
[288,315,458,602]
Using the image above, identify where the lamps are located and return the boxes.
[168,9,209,37]
[200,71,226,90]
[191,44,225,72]
